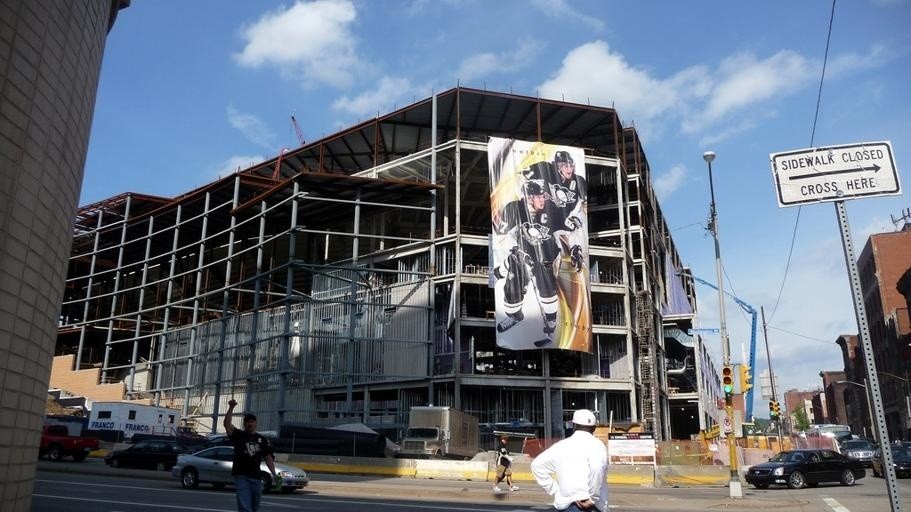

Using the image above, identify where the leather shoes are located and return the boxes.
[570,245,585,273]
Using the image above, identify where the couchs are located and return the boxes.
[722,366,734,395]
[768,400,780,420]
[739,364,754,394]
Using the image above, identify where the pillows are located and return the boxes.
[521,180,541,195]
[554,150,573,168]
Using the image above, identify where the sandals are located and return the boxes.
[806,425,852,449]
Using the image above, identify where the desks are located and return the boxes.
[703,150,728,363]
[836,377,877,443]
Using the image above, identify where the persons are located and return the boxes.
[529,409,611,512]
[490,433,521,493]
[492,149,588,296]
[223,398,282,511]
[492,180,584,349]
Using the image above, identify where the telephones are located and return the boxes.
[771,142,902,207]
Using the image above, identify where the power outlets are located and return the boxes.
[38,424,99,462]
[131,426,209,445]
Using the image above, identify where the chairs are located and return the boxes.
[543,311,557,332]
[492,485,501,492]
[497,307,524,332]
[508,486,519,491]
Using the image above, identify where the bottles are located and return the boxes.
[571,407,596,427]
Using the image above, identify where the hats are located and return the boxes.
[745,449,865,490]
[872,446,911,478]
[206,435,233,447]
[104,440,196,471]
[171,445,309,494]
[840,440,876,468]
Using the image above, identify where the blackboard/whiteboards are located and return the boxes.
[698,423,795,464]
